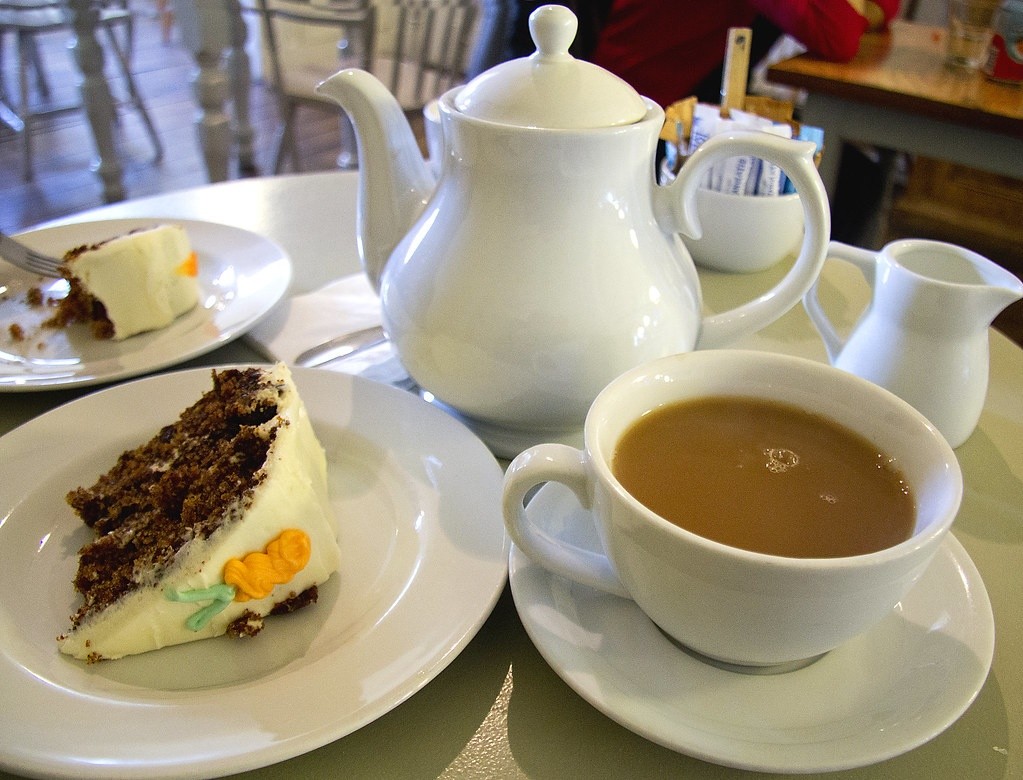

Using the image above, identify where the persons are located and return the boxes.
[495,0,901,164]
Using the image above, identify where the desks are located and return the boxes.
[767,19,1022,139]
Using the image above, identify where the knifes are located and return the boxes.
[294,324,387,369]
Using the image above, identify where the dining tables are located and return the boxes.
[0,169,1023,779]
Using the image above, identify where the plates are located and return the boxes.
[0,217,296,389]
[508,479,994,772]
[0,365,512,780]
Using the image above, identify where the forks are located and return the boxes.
[0,232,65,278]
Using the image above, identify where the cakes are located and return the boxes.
[28,223,198,341]
[58,362,342,667]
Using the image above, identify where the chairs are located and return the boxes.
[0,2,493,201]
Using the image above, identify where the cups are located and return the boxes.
[658,160,807,273]
[502,349,965,668]
[801,239,1023,450]
[942,2,1005,70]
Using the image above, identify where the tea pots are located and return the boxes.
[314,4,831,458]
